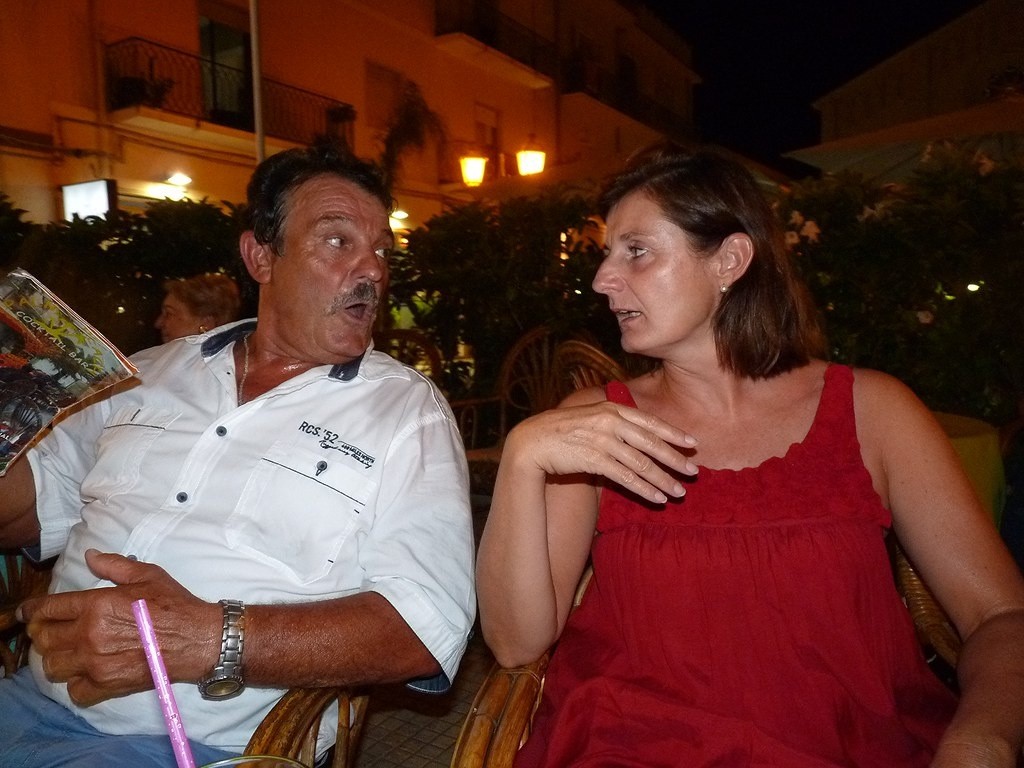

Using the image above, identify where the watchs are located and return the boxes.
[199,600,246,702]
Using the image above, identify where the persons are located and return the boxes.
[156,272,239,344]
[474,154,1024,768]
[0,149,476,767]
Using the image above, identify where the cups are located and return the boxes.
[200,755,307,768]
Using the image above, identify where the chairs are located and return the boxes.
[0,323,1024,768]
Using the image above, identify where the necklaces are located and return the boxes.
[238,331,253,404]
